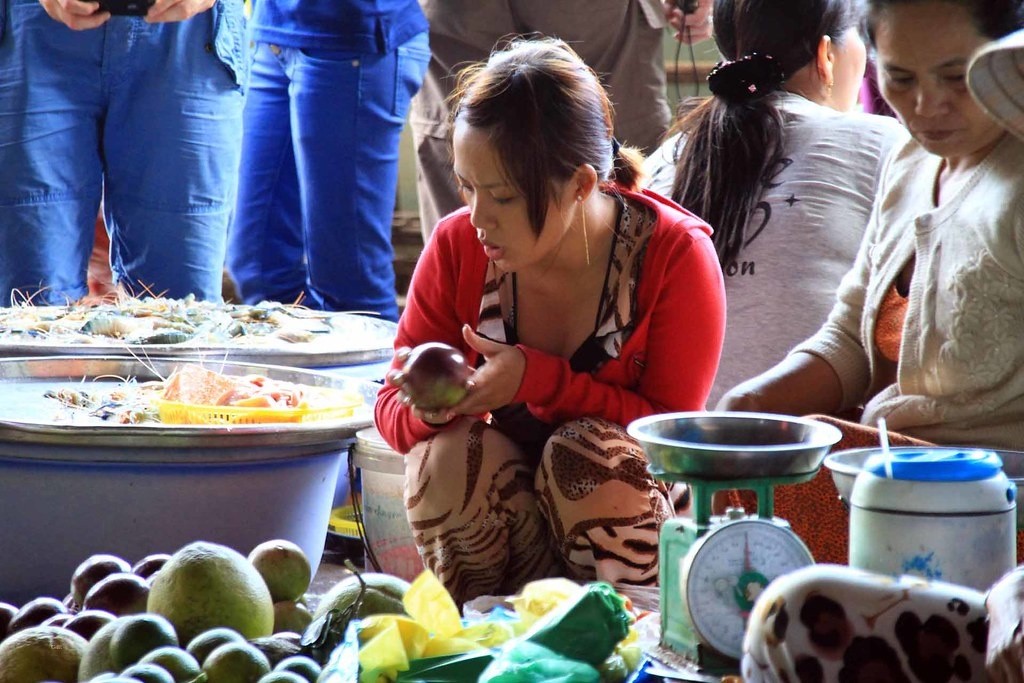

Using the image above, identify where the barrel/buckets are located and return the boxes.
[353,431,424,587]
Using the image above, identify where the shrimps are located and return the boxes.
[0,279,322,345]
[43,375,162,425]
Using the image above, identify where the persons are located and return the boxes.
[712,0,1024,559]
[410,0,714,247]
[629,0,910,416]
[374,39,728,612]
[226,0,430,326]
[0,0,250,307]
[741,559,1024,683]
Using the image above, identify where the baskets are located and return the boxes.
[156,395,303,426]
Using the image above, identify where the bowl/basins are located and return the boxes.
[2,302,399,382]
[2,355,385,609]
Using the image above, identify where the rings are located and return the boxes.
[707,16,713,24]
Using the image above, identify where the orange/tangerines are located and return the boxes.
[79,614,323,683]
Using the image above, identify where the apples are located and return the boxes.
[401,342,469,413]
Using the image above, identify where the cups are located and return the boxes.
[848,447,1017,592]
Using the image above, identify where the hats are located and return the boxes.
[967,30,1024,140]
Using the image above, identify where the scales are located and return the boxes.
[627,411,843,683]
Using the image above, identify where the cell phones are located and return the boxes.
[80,0,157,16]
